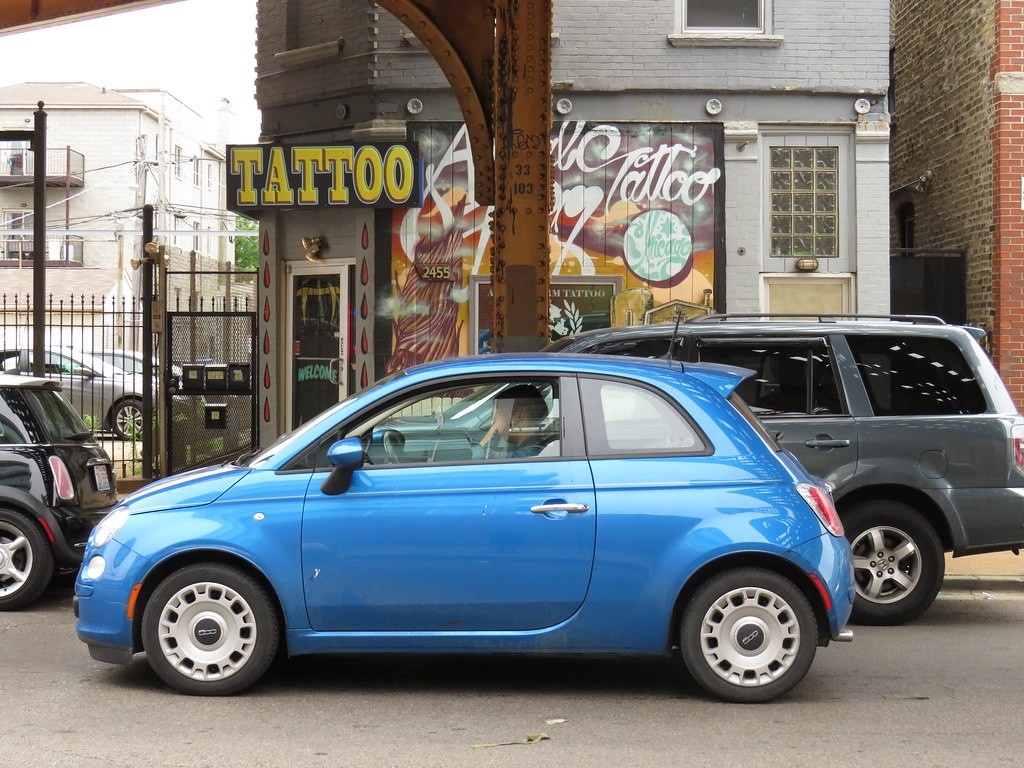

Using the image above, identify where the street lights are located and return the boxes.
[0,129,46,376]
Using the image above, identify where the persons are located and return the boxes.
[465,384,548,459]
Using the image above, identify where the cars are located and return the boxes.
[1,346,161,439]
[1,375,117,606]
[81,348,182,387]
[75,353,858,704]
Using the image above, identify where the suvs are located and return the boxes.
[373,313,1024,626]
